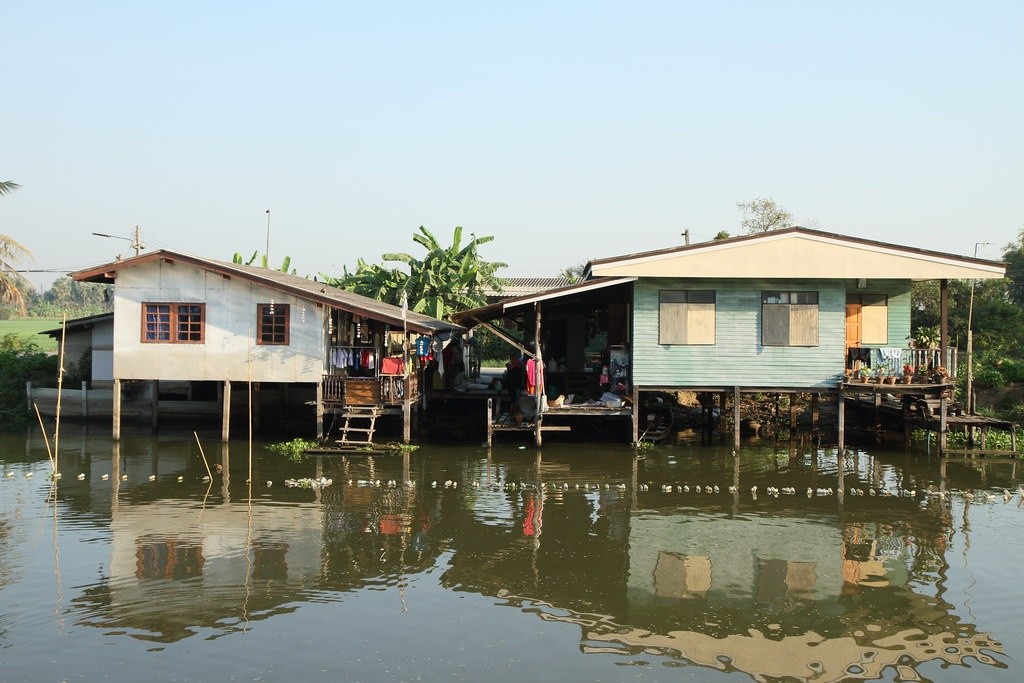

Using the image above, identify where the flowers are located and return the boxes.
[918,364,927,376]
[931,365,949,377]
[903,364,913,376]
[875,363,888,377]
[858,365,872,377]
[888,368,897,377]
[843,368,852,377]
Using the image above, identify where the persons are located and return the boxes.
[487,351,522,389]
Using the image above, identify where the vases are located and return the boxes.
[919,376,927,384]
[875,377,884,384]
[844,376,851,383]
[887,377,897,385]
[935,377,945,384]
[861,377,869,383]
[903,376,913,384]
